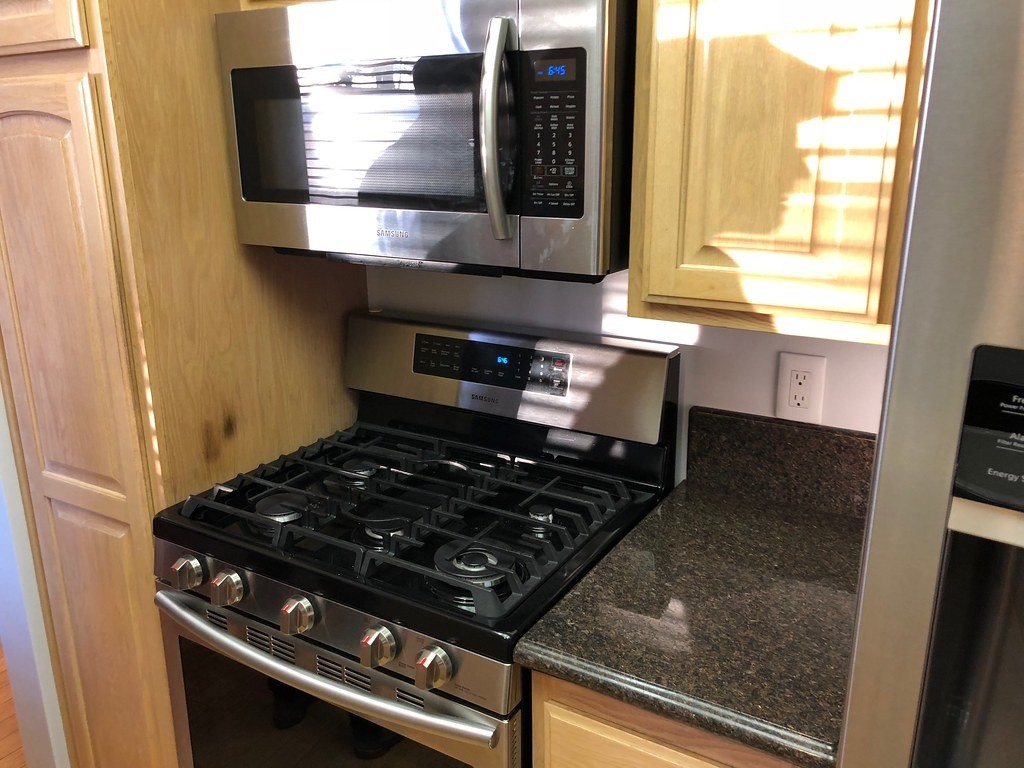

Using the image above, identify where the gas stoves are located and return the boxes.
[152,307,682,714]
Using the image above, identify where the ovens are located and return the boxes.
[152,579,533,768]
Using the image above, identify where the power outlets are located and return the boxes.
[775,351,827,425]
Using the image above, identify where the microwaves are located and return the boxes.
[215,1,637,284]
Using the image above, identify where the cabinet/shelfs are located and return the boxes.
[625,0,935,328]
[531,669,800,768]
[0,0,358,768]
